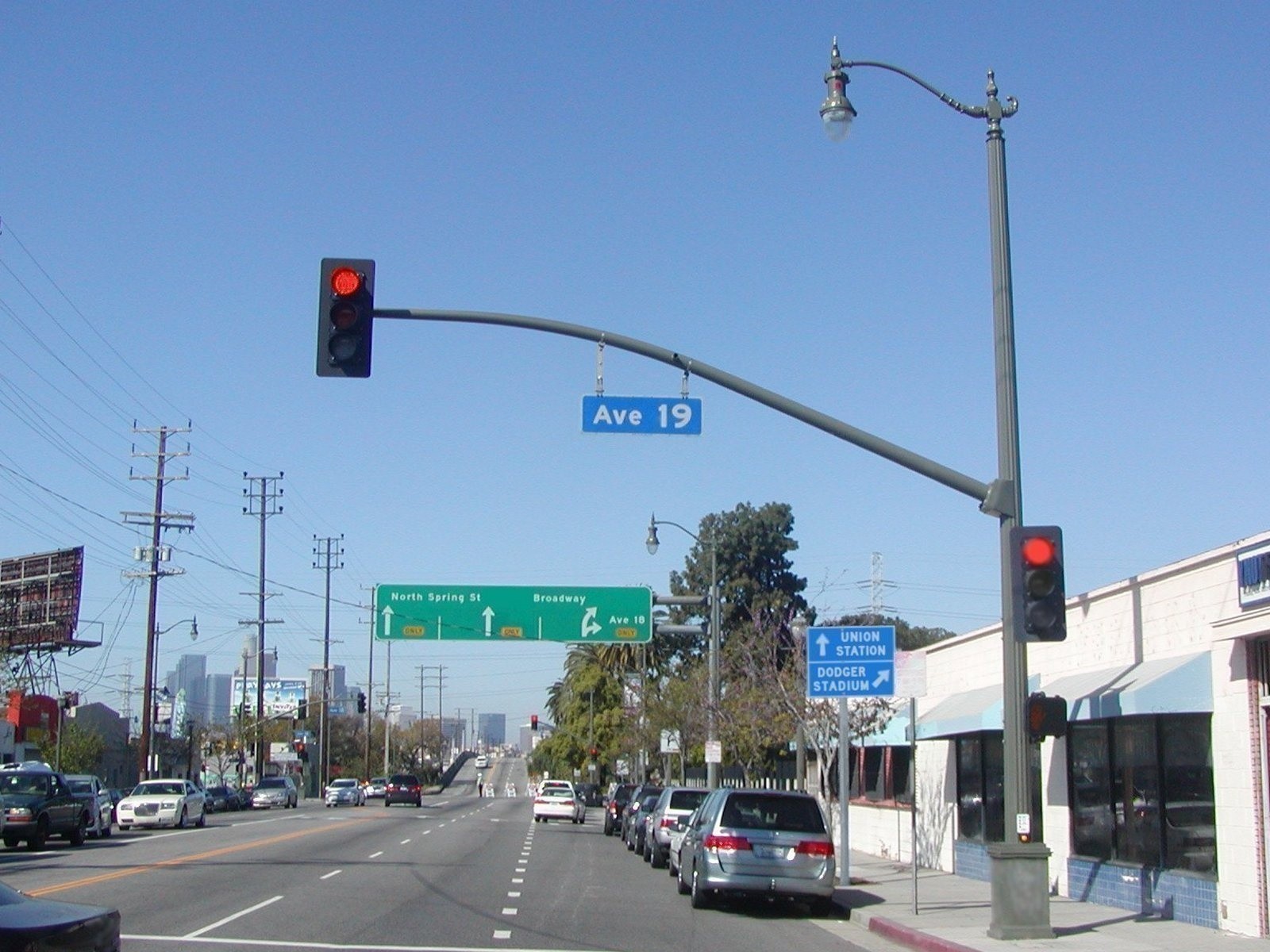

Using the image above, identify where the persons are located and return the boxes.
[476,773,484,798]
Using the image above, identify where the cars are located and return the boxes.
[0,761,423,838]
[535,780,834,913]
[0,879,123,952]
[475,755,488,769]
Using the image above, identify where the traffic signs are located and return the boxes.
[375,584,656,645]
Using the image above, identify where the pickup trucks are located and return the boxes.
[1,769,95,850]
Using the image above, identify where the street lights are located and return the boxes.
[149,615,279,779]
[819,37,1054,941]
[644,510,723,788]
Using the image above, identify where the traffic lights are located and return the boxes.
[357,693,366,713]
[1009,525,1067,643]
[297,743,307,760]
[590,747,596,763]
[312,257,377,379]
[531,715,538,731]
